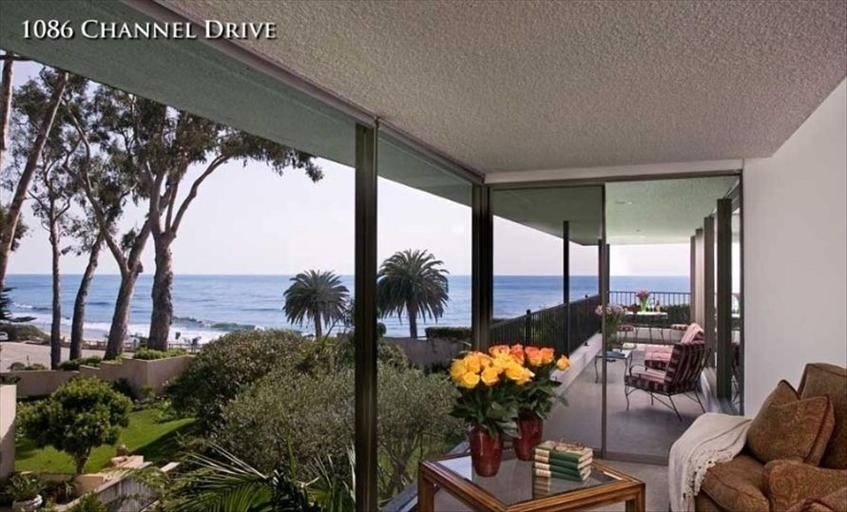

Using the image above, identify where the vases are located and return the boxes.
[463,423,503,475]
[636,297,648,312]
[507,409,544,462]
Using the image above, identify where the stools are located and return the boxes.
[614,309,689,345]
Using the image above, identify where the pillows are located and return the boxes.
[740,377,837,472]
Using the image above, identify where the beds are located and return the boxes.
[412,440,649,512]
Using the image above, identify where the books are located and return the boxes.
[532,476,592,499]
[533,439,594,481]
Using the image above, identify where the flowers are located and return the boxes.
[633,289,651,312]
[439,346,537,440]
[486,342,572,425]
[592,305,627,350]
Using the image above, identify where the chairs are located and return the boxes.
[666,363,847,512]
[643,320,705,372]
[621,339,714,423]
